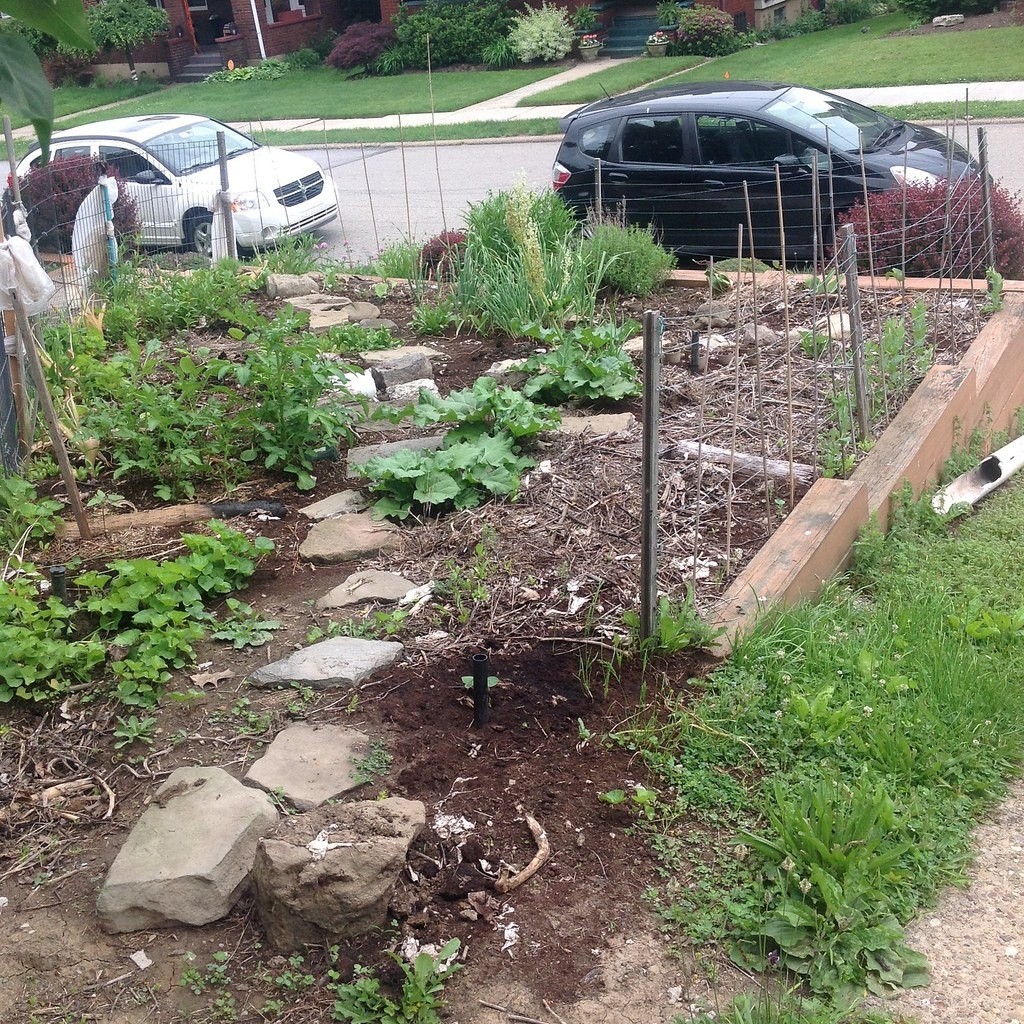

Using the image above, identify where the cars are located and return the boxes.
[6,115,340,258]
[551,80,995,270]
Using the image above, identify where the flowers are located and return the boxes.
[580,34,599,46]
[648,32,668,43]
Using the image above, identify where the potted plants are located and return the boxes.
[571,5,599,30]
[658,1,680,44]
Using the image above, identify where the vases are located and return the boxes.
[578,43,599,62]
[646,39,670,57]
